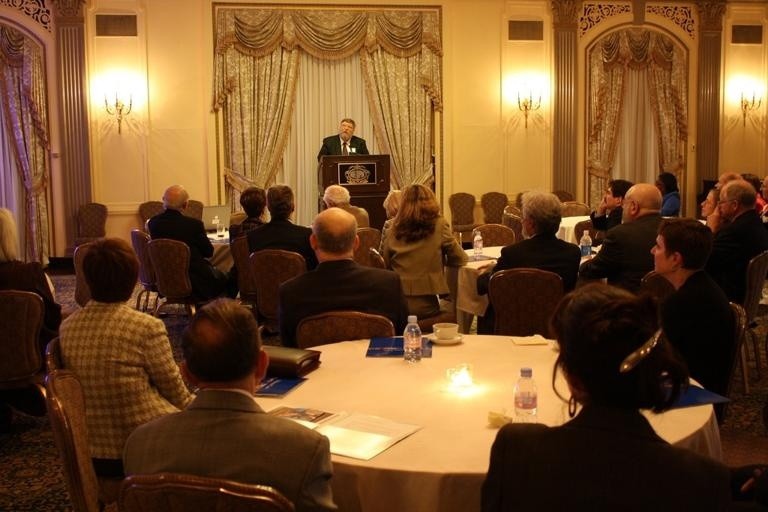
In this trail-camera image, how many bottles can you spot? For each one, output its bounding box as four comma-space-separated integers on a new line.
403, 315, 422, 365
474, 231, 484, 259
580, 231, 592, 262
514, 368, 539, 425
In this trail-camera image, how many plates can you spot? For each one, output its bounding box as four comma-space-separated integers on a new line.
427, 332, 464, 344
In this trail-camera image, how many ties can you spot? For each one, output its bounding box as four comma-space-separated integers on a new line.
342, 142, 348, 155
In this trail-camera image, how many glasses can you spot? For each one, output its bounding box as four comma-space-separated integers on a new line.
718, 199, 733, 207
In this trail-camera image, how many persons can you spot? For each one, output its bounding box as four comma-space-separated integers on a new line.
56, 170, 470, 511
0, 205, 64, 354
317, 118, 369, 162
476, 170, 766, 512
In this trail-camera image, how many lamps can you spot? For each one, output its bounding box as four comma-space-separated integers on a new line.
105, 77, 133, 133
741, 87, 762, 127
517, 77, 541, 128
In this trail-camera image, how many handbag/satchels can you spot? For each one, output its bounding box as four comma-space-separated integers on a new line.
135, 307, 192, 356
261, 346, 323, 376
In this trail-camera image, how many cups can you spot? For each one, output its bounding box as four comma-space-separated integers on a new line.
432, 323, 459, 338
216, 224, 225, 237
